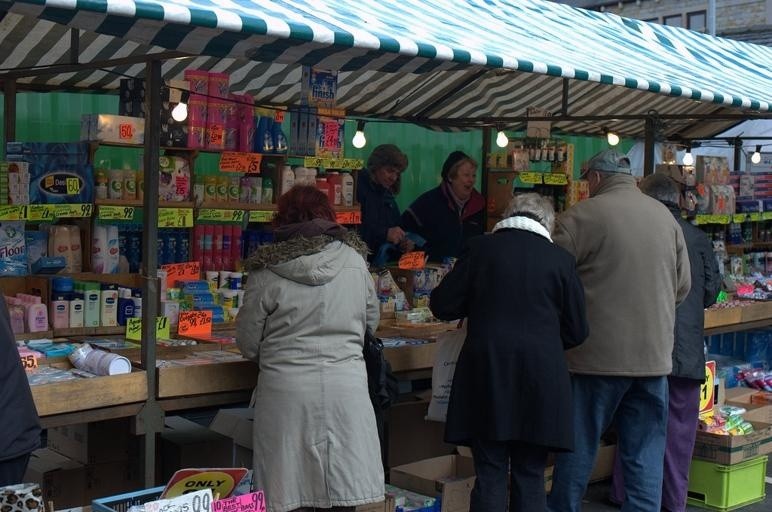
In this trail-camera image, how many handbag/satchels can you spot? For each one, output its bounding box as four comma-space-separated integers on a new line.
362, 320, 403, 416
420, 314, 480, 424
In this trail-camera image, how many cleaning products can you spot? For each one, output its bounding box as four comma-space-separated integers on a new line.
0, 220, 65, 276
159, 155, 191, 202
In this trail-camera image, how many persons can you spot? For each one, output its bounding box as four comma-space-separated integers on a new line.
428, 193, 589, 512
352, 144, 428, 271
608, 174, 722, 512
399, 151, 487, 263
551, 148, 692, 512
234, 184, 386, 512
0, 286, 45, 486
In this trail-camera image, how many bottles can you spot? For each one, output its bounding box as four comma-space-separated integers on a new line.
120, 226, 243, 272
205, 271, 246, 323
281, 163, 353, 207
97, 168, 273, 204
6, 277, 142, 335
742, 246, 772, 275
39, 224, 121, 274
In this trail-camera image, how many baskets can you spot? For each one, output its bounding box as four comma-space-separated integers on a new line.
90, 483, 167, 511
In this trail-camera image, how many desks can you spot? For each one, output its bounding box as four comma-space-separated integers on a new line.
0, 277, 770, 493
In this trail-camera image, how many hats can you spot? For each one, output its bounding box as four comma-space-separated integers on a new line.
366, 141, 410, 175
439, 149, 470, 184
576, 147, 634, 181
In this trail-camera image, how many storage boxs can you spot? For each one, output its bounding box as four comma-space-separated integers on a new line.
17, 372, 772, 512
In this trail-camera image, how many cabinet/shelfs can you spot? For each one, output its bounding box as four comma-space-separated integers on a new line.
1, 132, 372, 343
483, 136, 575, 237
629, 172, 771, 297
